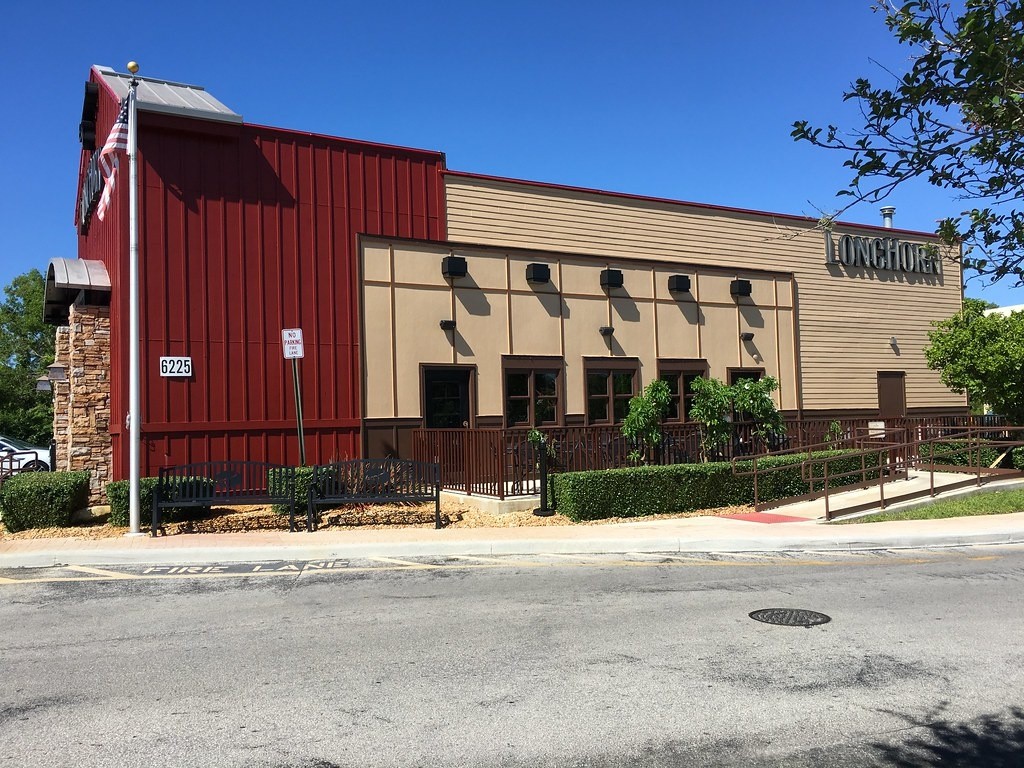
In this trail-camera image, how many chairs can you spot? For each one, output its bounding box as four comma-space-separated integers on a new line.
148, 459, 298, 534
307, 456, 442, 530
488, 432, 704, 495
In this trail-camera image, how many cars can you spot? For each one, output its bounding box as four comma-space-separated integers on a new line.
0, 437, 54, 477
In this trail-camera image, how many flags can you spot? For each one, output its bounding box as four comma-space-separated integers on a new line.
96, 92, 131, 220
